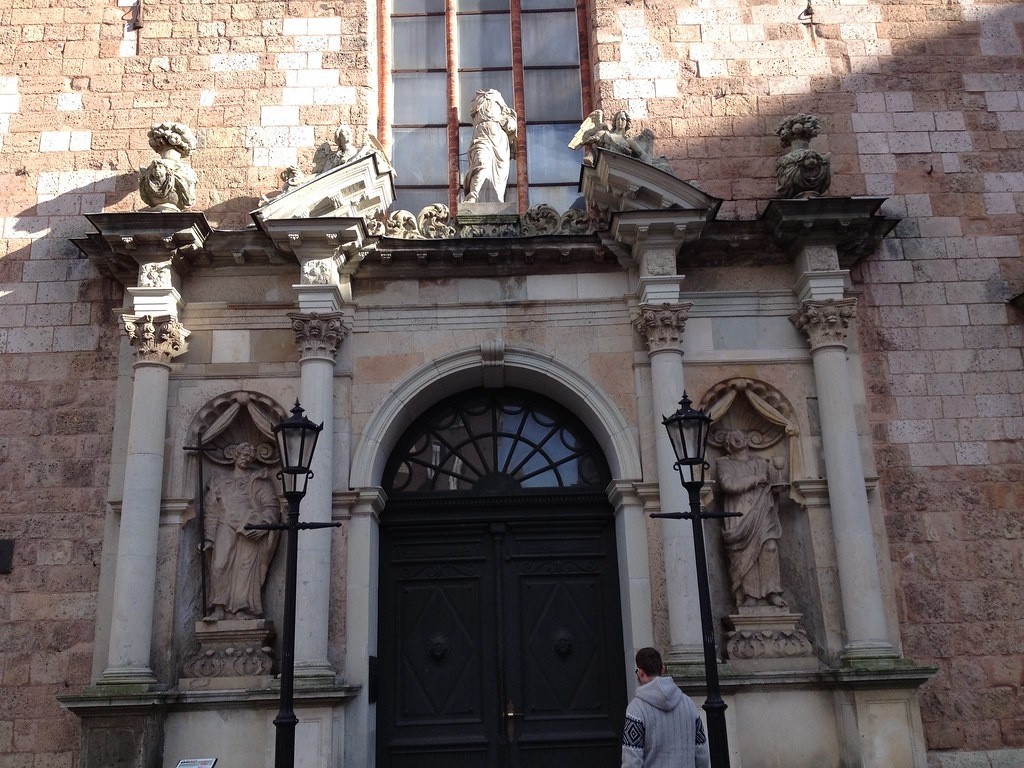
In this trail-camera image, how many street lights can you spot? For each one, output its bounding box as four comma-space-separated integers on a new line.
649, 391, 744, 767
244, 398, 342, 767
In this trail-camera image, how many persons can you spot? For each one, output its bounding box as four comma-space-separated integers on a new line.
290, 124, 396, 231
145, 160, 167, 184
622, 647, 710, 768
463, 89, 517, 203
716, 430, 790, 607
197, 442, 280, 622
584, 110, 643, 167
798, 149, 820, 177
